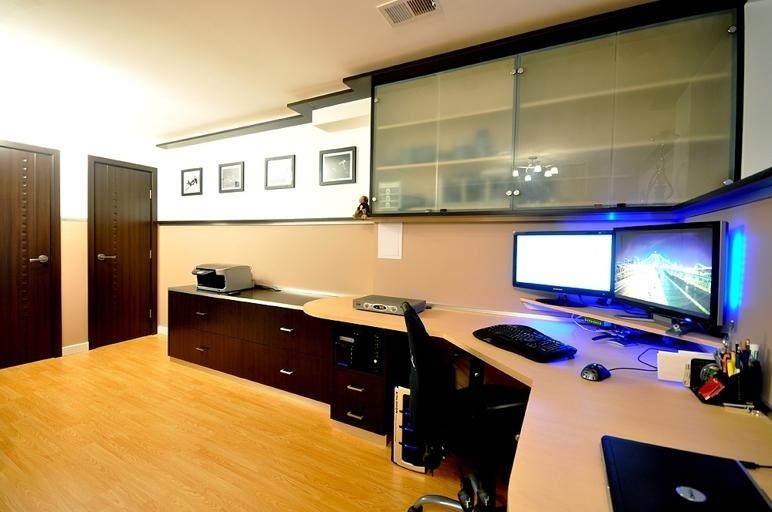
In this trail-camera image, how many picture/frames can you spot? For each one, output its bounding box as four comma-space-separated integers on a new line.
319, 145, 356, 186
180, 167, 202, 196
218, 161, 244, 193
264, 154, 295, 190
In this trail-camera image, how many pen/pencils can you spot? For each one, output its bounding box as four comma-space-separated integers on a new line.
720, 320, 759, 378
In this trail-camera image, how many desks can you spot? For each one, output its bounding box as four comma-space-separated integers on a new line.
303, 295, 771, 511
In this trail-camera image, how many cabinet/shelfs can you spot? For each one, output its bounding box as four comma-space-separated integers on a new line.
168, 282, 333, 405
369, 9, 738, 217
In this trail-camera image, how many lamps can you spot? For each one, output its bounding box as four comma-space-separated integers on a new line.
512, 155, 559, 182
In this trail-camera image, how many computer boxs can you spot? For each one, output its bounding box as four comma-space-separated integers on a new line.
393, 378, 430, 474
498, 388, 530, 483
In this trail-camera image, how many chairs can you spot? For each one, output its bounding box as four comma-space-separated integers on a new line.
400, 301, 529, 511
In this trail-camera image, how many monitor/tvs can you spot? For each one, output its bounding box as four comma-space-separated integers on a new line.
612, 221, 726, 329
513, 231, 614, 307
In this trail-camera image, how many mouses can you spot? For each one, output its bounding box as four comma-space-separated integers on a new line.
581, 363, 610, 381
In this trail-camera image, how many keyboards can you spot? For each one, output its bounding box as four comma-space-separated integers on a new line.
473, 324, 577, 362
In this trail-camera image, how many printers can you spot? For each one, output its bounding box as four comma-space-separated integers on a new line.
192, 263, 253, 293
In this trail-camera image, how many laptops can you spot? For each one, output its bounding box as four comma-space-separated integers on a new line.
601, 436, 772, 512
